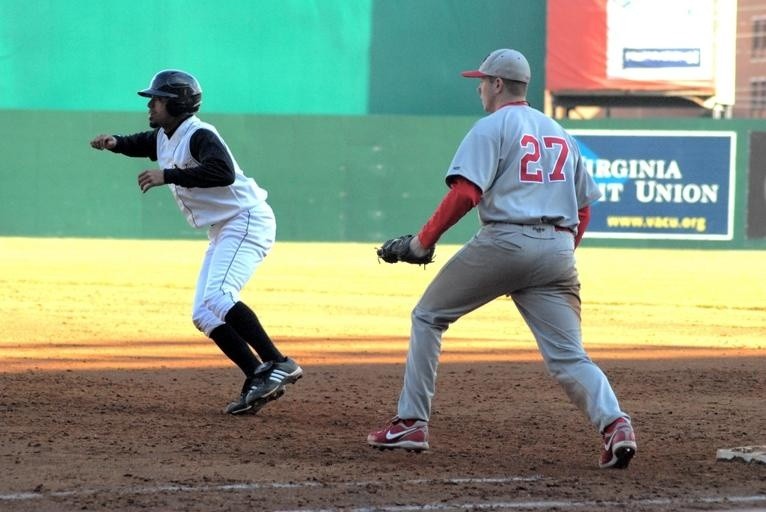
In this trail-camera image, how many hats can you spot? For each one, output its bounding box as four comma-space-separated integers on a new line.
137, 70, 201, 116
461, 49, 530, 85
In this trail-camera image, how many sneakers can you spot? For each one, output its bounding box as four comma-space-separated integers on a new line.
245, 354, 303, 405
367, 415, 430, 450
226, 370, 286, 415
598, 417, 637, 469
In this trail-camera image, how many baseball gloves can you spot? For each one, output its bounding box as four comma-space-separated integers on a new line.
377, 235, 434, 263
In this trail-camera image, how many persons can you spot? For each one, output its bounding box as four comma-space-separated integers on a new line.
366, 48, 637, 470
87, 71, 305, 416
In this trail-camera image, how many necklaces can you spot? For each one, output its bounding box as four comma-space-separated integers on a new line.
521, 99, 532, 107
